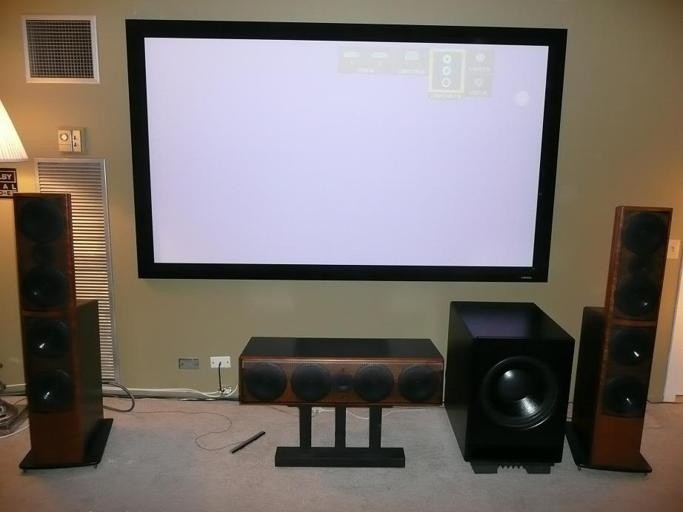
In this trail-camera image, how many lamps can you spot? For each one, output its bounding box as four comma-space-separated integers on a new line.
1, 98, 29, 162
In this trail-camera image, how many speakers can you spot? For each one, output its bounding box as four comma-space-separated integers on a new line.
571, 205, 675, 469
444, 301, 575, 476
239, 335, 443, 408
13, 191, 104, 465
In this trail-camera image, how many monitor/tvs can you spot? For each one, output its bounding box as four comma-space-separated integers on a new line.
122, 17, 569, 285
